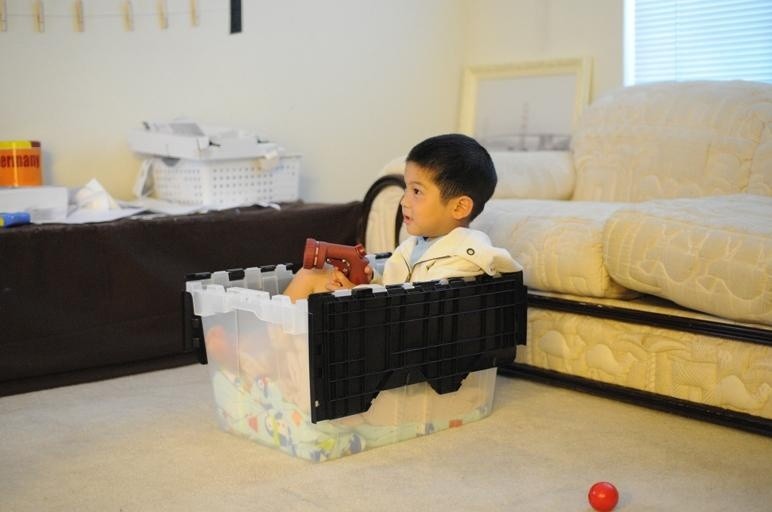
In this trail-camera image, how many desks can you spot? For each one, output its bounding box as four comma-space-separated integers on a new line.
1, 200, 366, 398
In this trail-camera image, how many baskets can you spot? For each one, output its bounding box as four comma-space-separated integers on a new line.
154, 153, 303, 206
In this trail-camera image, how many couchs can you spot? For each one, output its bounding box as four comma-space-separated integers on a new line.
362, 78, 772, 442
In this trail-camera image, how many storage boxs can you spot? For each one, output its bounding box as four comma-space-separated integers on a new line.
182, 259, 529, 464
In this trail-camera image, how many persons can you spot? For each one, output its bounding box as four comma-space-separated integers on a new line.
280, 131, 524, 306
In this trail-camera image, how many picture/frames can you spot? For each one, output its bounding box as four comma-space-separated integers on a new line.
458, 54, 594, 152
